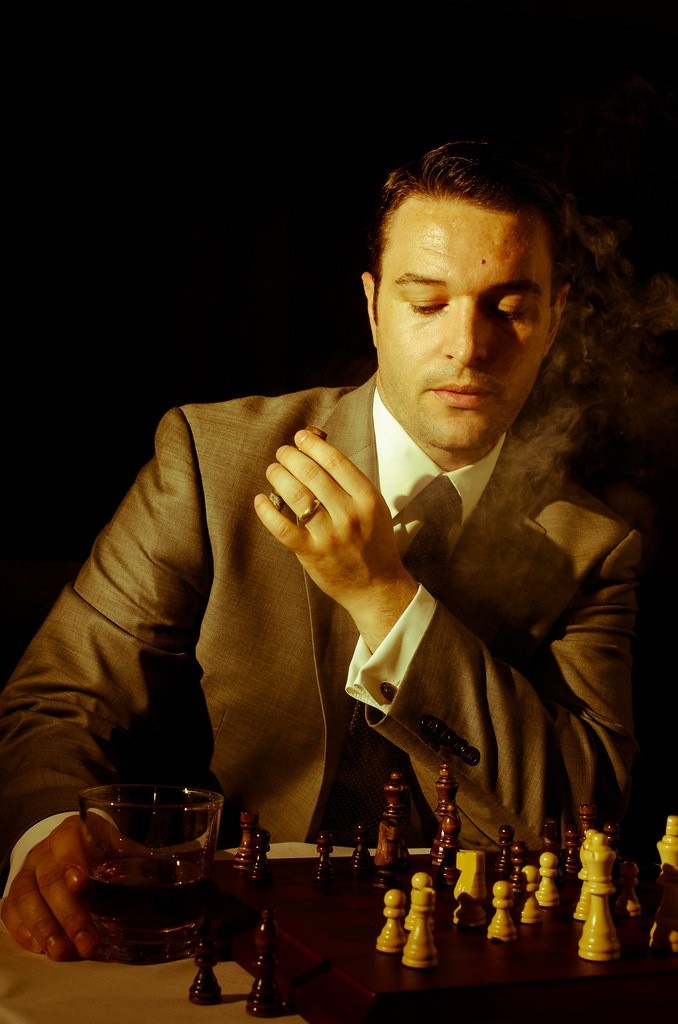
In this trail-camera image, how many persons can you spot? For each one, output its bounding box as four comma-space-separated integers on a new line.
0, 143, 643, 961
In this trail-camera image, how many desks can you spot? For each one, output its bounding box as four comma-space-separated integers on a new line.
0, 842, 432, 1024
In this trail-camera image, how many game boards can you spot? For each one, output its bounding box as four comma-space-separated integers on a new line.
188, 847, 678, 1024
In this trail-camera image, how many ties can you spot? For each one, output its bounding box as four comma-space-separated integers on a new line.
321, 476, 463, 849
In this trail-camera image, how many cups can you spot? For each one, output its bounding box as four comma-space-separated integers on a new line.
78, 783, 224, 965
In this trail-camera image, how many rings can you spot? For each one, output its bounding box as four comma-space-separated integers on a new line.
299, 499, 320, 524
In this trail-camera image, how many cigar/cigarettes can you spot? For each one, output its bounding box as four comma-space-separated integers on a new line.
269, 426, 328, 512
